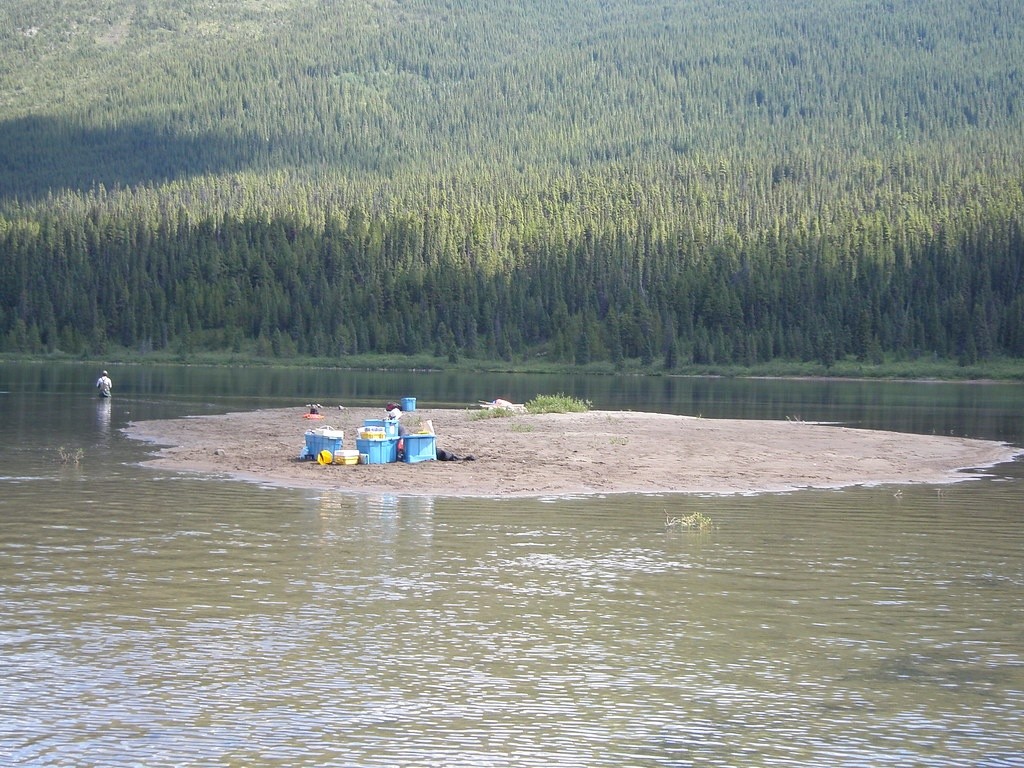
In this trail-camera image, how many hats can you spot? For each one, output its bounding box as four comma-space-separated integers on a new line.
103, 371, 107, 375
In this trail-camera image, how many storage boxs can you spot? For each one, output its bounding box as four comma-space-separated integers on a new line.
358, 454, 370, 465
365, 419, 400, 436
356, 438, 388, 466
386, 436, 401, 463
401, 397, 417, 411
316, 429, 343, 436
334, 456, 359, 466
305, 434, 344, 461
310, 409, 317, 414
334, 450, 360, 457
401, 435, 438, 464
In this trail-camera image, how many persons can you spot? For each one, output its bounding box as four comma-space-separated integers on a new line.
96, 370, 112, 397
